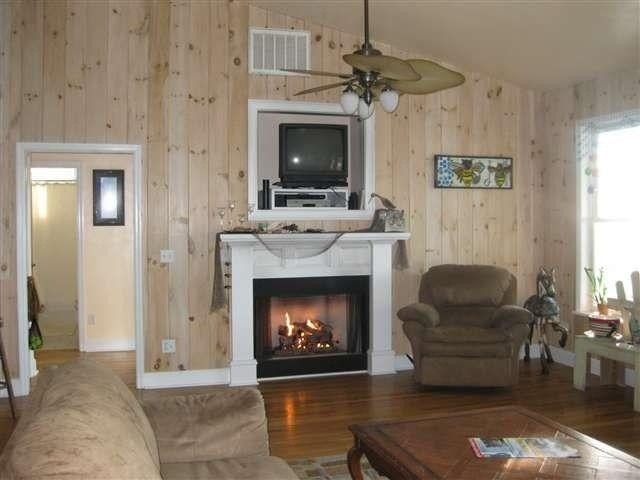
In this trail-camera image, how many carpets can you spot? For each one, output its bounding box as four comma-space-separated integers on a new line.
287, 453, 391, 480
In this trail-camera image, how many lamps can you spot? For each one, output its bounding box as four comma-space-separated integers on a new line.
340, 73, 398, 120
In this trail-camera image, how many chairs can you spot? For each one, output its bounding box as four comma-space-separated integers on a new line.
397, 272, 534, 390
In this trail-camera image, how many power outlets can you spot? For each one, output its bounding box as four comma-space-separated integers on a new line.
162, 339, 177, 354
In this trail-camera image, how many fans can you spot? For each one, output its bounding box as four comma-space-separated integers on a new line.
281, 0, 465, 96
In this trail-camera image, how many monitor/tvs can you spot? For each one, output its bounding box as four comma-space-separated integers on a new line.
279, 123, 348, 182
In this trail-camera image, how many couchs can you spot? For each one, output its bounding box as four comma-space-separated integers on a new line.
1, 363, 300, 480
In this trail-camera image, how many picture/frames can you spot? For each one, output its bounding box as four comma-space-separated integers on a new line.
92, 170, 124, 226
435, 154, 513, 189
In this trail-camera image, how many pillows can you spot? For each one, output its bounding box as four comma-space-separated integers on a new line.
428, 265, 511, 307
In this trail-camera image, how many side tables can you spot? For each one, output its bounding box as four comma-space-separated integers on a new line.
573, 335, 640, 412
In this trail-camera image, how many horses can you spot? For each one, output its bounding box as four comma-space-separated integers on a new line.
522, 264, 570, 372
